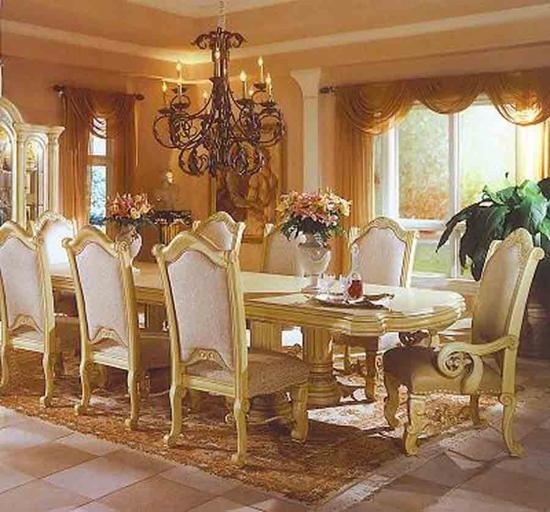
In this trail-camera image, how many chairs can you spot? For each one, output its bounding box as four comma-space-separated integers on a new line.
189, 209, 247, 257
150, 227, 313, 469
381, 225, 545, 459
26, 209, 80, 264
1, 218, 111, 408
258, 222, 332, 277
60, 224, 172, 430
330, 215, 421, 402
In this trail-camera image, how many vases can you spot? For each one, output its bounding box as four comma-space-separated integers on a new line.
292, 233, 333, 298
113, 222, 145, 275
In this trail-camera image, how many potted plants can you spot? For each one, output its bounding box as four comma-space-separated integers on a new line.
435, 171, 550, 360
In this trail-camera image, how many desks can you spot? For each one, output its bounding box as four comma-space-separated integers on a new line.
48, 257, 466, 409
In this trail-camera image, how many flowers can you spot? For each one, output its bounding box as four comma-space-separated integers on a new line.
103, 192, 156, 230
274, 187, 353, 242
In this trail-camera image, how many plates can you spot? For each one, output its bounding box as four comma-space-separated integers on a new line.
313, 291, 395, 309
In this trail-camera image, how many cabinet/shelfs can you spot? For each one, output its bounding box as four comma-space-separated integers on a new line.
0, 96, 65, 235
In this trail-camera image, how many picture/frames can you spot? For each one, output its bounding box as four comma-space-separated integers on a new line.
211, 124, 287, 243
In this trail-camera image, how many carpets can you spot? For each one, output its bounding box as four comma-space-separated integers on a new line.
0, 324, 525, 505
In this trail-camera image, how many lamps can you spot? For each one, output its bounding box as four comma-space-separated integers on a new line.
153, 0, 286, 179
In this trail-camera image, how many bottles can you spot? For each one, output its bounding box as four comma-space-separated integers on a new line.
348, 276, 363, 298
